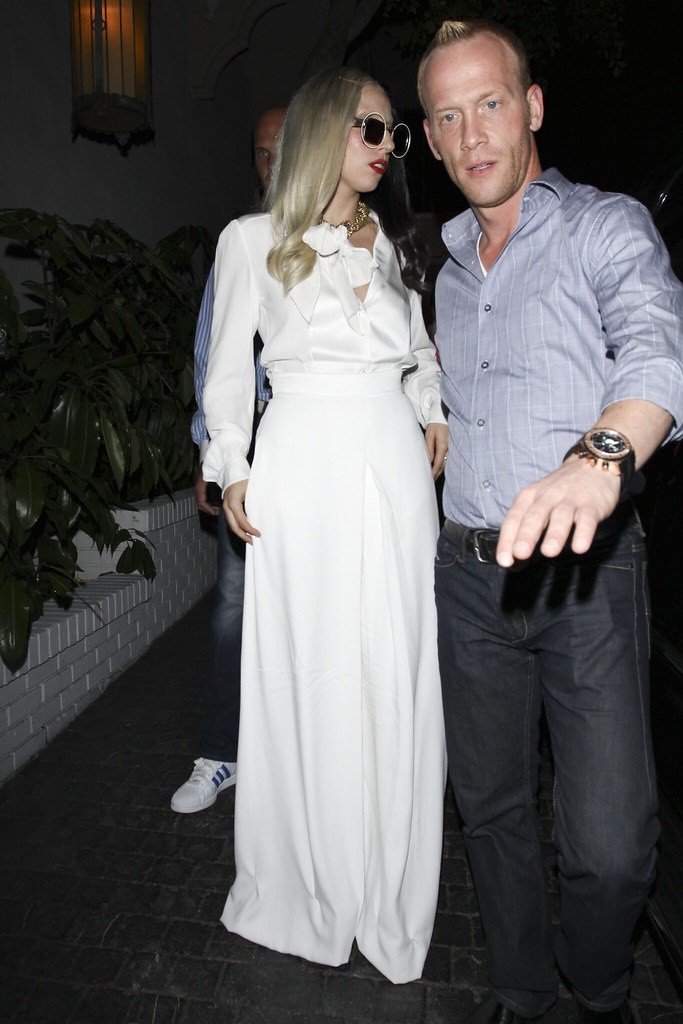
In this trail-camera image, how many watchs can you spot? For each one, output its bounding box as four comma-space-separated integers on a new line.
563, 427, 635, 485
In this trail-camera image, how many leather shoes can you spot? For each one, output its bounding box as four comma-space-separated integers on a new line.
489, 997, 561, 1024
578, 999, 635, 1024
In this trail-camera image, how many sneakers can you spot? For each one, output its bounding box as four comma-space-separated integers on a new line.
169, 757, 236, 813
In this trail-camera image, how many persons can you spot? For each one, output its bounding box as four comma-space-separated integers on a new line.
201, 61, 451, 985
416, 19, 683, 1024
170, 96, 294, 814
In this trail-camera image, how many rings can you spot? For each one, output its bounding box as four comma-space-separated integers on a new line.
444, 457, 447, 460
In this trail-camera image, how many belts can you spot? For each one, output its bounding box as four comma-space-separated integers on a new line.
440, 504, 630, 564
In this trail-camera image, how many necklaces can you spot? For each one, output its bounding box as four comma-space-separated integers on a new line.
309, 201, 369, 243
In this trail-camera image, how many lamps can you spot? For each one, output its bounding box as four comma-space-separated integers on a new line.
67, 0, 156, 150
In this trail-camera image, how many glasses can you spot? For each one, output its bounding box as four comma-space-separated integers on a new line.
351, 112, 412, 159
